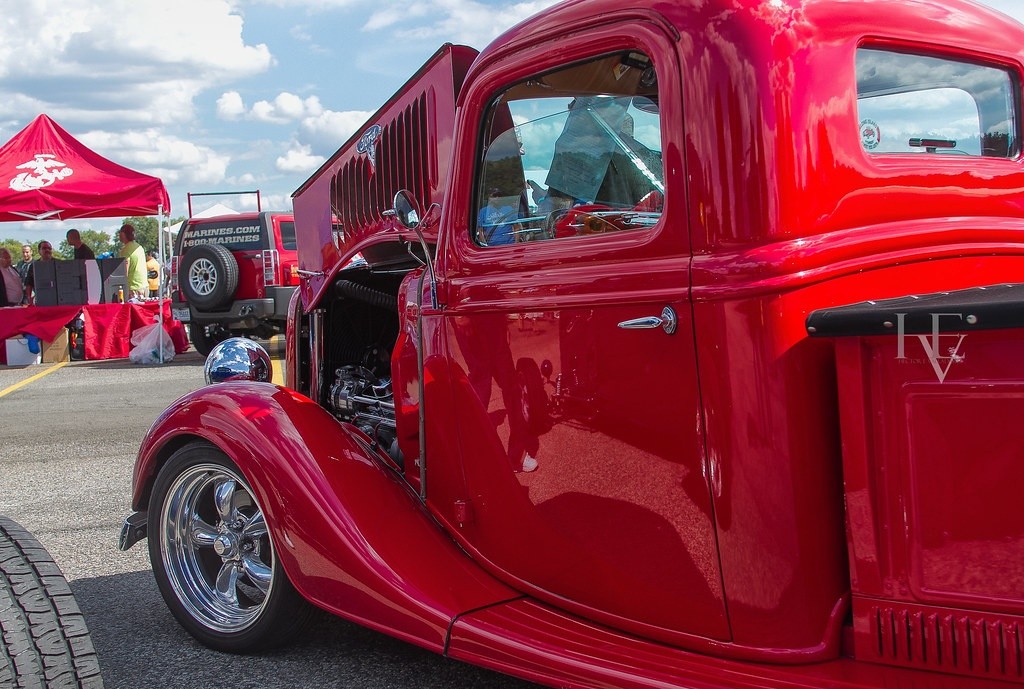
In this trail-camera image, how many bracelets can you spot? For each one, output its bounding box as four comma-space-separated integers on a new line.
535, 195, 544, 203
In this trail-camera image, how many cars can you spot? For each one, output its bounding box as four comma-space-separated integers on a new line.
118, 1, 1024, 689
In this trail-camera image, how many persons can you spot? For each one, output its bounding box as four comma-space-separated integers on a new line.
522, 101, 663, 215
476, 187, 521, 245
0, 224, 170, 308
456, 313, 538, 474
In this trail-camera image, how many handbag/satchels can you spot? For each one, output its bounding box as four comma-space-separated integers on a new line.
148, 271, 158, 279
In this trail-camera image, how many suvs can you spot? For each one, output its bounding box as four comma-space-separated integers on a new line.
172, 211, 344, 358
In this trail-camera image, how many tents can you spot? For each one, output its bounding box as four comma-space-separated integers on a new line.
164, 204, 241, 234
0, 113, 173, 364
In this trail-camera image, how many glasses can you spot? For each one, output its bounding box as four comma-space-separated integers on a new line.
41, 247, 52, 250
0, 256, 11, 261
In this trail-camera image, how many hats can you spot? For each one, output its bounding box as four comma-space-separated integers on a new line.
121, 224, 136, 240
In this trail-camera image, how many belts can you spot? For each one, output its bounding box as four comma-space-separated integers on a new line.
8, 302, 21, 306
